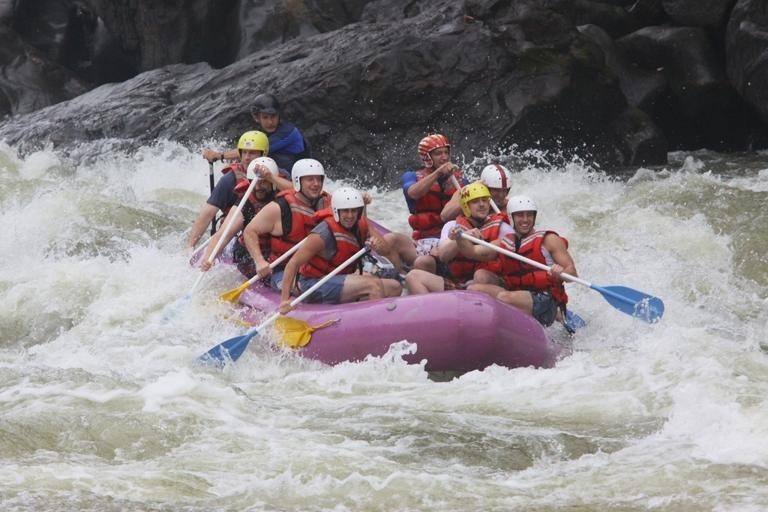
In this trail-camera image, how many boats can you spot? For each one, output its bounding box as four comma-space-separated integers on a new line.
201, 217, 565, 373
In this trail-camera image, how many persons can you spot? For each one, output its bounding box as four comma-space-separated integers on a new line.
202, 92, 307, 180
220, 151, 225, 163
240, 156, 375, 281
179, 130, 293, 253
199, 156, 290, 287
385, 130, 579, 329
278, 185, 402, 318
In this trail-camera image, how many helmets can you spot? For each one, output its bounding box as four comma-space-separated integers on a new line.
237, 130, 270, 160
246, 156, 280, 192
331, 187, 365, 223
249, 92, 281, 118
480, 163, 513, 197
458, 182, 492, 219
291, 158, 325, 197
506, 194, 538, 229
417, 134, 451, 169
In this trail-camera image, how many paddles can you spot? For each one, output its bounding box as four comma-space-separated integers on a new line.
461, 233, 664, 331
200, 238, 369, 369
